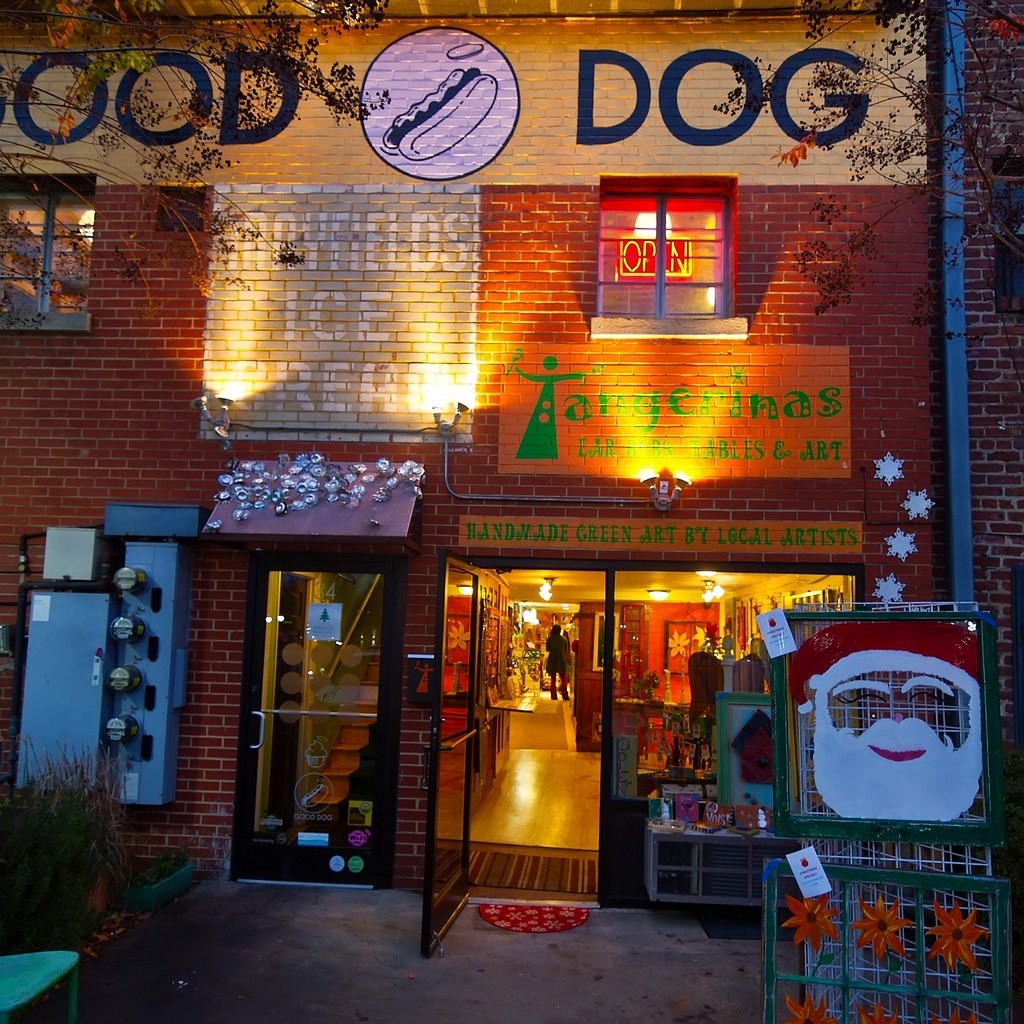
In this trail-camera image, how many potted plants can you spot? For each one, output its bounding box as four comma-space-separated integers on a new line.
127, 845, 193, 912
13, 731, 131, 914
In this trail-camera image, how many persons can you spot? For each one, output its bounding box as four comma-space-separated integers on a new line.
546, 625, 570, 701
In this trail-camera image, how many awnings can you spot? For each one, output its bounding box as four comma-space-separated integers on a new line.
201, 457, 424, 550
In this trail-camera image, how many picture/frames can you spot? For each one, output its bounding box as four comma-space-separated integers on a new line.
663, 620, 713, 675
594, 611, 619, 672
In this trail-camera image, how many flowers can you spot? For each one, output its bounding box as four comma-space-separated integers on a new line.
855, 1000, 899, 1024
850, 893, 912, 963
931, 1006, 977, 1024
781, 991, 839, 1024
924, 898, 990, 973
779, 893, 838, 954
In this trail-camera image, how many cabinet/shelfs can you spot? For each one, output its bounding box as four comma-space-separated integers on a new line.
644, 819, 800, 908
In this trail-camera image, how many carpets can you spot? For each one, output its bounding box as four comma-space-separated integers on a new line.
469, 850, 598, 894
478, 903, 591, 933
699, 912, 795, 941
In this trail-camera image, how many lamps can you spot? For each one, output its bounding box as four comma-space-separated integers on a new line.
701, 580, 724, 603
538, 578, 555, 602
647, 589, 671, 601
637, 468, 691, 511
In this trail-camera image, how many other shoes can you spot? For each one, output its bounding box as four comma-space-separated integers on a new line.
558, 687, 570, 700
552, 692, 557, 699
